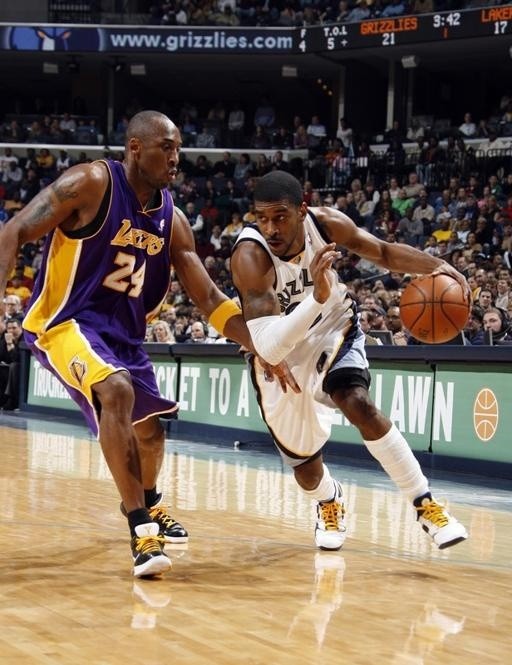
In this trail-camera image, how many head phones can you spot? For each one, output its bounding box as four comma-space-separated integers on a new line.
198, 320, 209, 336
481, 309, 512, 334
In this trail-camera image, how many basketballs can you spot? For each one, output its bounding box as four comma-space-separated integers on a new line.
399, 274, 469, 344
474, 389, 499, 441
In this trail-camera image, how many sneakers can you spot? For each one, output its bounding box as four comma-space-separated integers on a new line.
314, 479, 347, 550
130, 521, 172, 578
414, 492, 467, 550
121, 491, 190, 544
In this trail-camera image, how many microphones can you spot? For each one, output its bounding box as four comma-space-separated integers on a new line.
191, 335, 206, 340
493, 326, 511, 340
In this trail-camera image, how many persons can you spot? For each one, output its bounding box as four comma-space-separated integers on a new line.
1, 108, 136, 347
228, 169, 478, 556
132, 2, 435, 28
144, 97, 512, 346
0, 109, 303, 581
